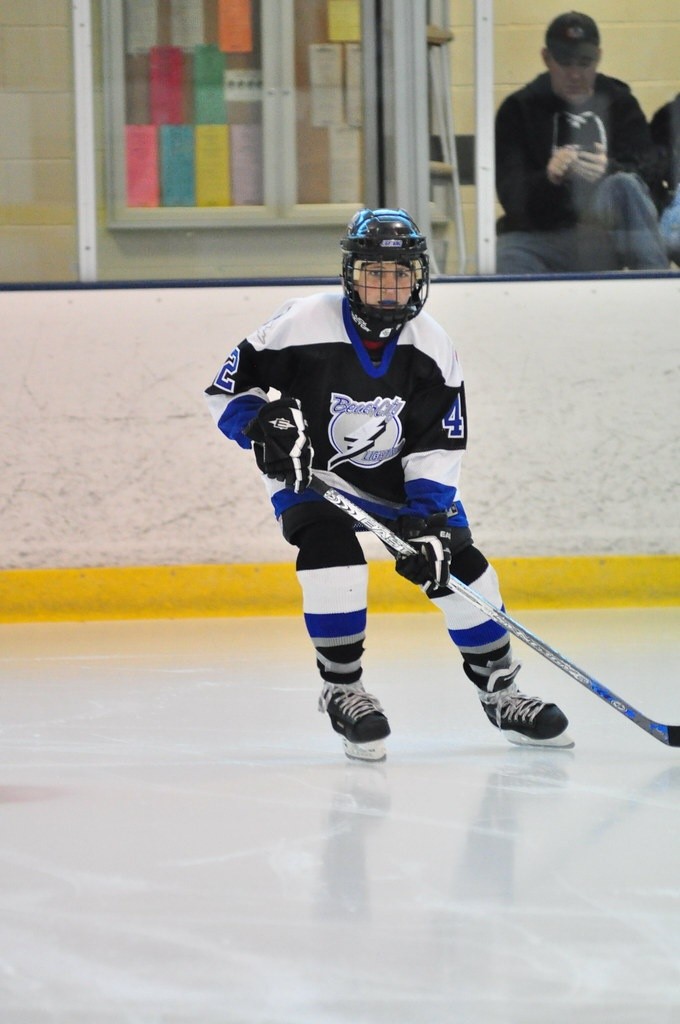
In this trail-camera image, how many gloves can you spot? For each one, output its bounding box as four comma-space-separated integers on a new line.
393, 514, 456, 597
240, 399, 314, 497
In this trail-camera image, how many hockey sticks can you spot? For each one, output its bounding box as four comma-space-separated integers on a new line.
305, 474, 678, 750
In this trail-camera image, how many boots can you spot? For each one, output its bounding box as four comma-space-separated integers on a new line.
463, 658, 577, 749
316, 678, 391, 763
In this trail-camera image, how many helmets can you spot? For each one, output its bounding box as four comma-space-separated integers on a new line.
338, 208, 431, 340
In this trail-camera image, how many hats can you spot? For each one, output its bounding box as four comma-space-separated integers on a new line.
545, 12, 599, 62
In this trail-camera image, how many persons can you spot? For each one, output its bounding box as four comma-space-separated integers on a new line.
494, 12, 680, 276
205, 210, 576, 761
